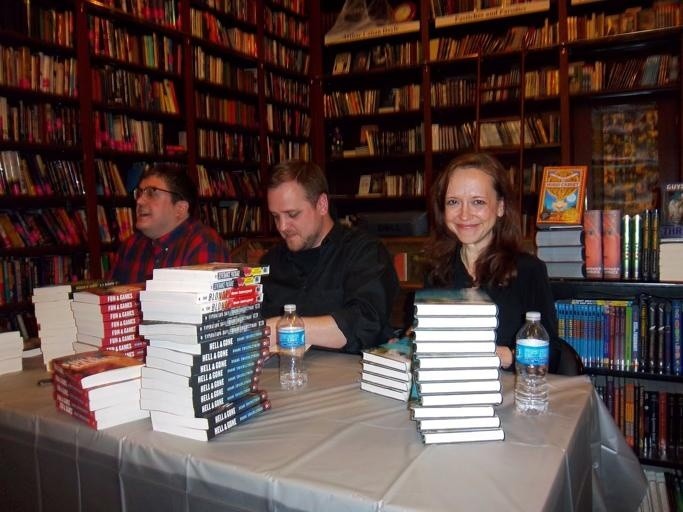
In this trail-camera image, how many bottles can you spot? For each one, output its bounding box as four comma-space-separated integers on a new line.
515, 312, 549, 416
276, 304, 306, 390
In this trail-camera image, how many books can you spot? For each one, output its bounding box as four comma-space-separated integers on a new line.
567, 0, 683, 93
587, 374, 683, 512
658, 182, 683, 282
584, 209, 659, 280
412, 289, 504, 444
1, 262, 272, 441
2, 0, 313, 111
428, 0, 561, 150
2, 202, 137, 343
554, 293, 683, 376
359, 337, 413, 400
324, 0, 424, 198
1, 98, 312, 200
534, 164, 587, 278
202, 202, 285, 266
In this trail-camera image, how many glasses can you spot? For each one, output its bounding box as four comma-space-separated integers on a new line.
133, 186, 184, 201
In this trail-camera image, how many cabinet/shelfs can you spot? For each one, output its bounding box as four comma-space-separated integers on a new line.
264, 0, 322, 168
190, 1, 270, 257
561, 0, 683, 210
546, 274, 683, 512
422, 0, 565, 237
319, 0, 425, 230
0, 0, 100, 340
85, 1, 202, 280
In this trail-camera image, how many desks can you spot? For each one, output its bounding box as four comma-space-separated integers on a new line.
1, 342, 648, 512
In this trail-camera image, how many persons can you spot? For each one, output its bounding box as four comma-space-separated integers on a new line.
103, 163, 229, 286
255, 159, 401, 361
389, 151, 558, 376
